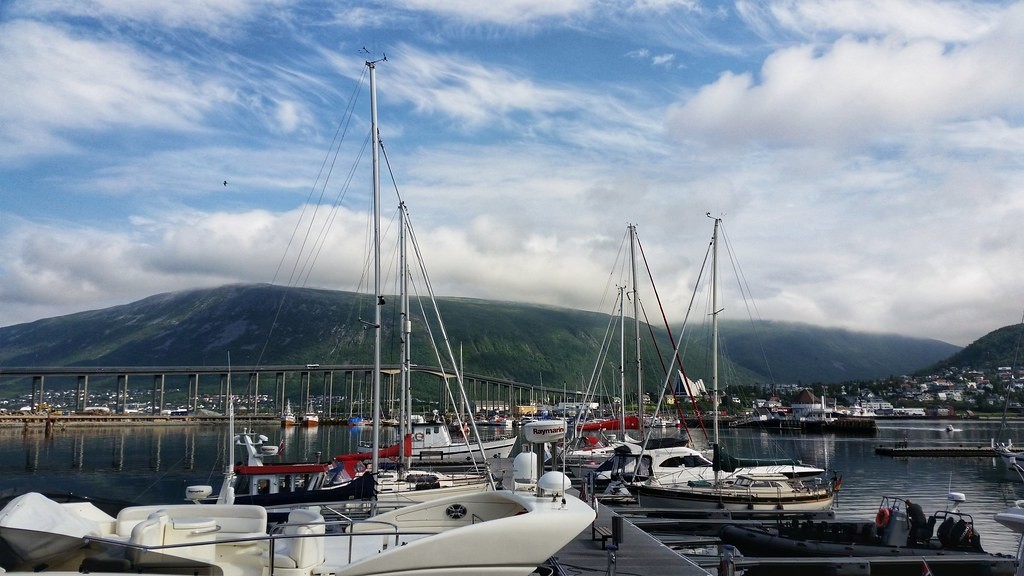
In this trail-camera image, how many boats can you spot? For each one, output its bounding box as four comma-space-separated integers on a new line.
719, 471, 986, 555
946, 424, 952, 432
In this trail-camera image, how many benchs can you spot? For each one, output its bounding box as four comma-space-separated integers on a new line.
115, 504, 267, 546
592, 526, 614, 550
258, 509, 326, 576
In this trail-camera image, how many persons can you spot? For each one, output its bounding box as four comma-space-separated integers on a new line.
906, 500, 926, 536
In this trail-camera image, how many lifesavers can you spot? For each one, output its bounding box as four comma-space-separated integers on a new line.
414, 432, 425, 441
875, 507, 889, 528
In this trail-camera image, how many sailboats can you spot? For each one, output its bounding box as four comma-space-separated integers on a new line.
0, 54, 595, 575
626, 211, 837, 520
561, 219, 826, 478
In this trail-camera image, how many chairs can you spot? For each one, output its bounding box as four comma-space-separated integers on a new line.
920, 516, 966, 547
787, 518, 878, 544
90, 509, 169, 553
